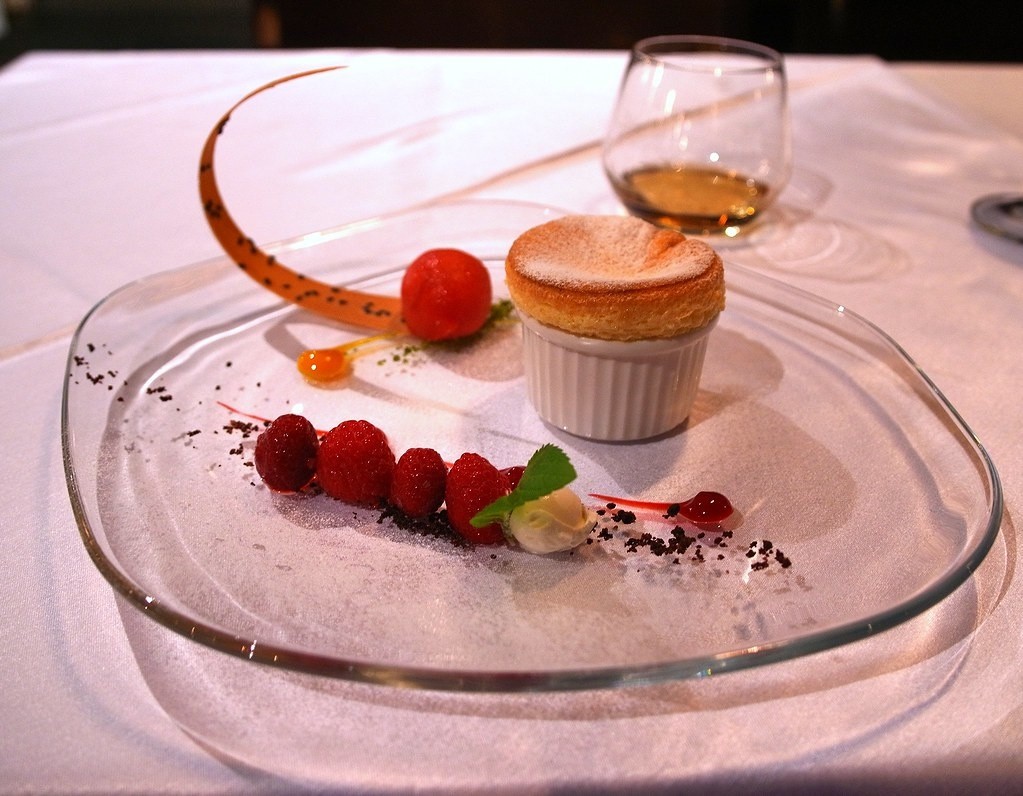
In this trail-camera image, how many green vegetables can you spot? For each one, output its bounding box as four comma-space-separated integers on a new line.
469, 444, 578, 541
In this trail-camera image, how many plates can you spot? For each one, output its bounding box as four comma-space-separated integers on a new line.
63, 198, 1004, 693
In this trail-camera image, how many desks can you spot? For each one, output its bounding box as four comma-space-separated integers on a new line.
2, 49, 1022, 796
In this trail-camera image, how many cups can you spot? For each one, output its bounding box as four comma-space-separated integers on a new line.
603, 36, 794, 236
509, 295, 721, 441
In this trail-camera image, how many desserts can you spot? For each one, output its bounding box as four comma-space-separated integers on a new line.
197, 66, 510, 343
506, 214, 725, 443
